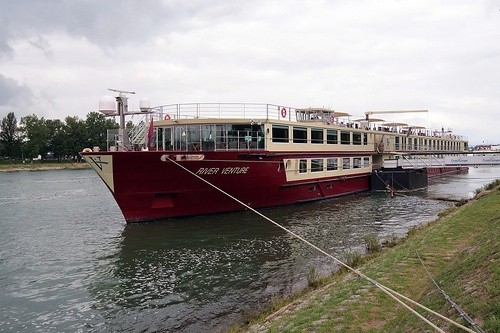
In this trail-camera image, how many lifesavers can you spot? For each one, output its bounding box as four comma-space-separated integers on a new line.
281, 108, 286, 117
164, 115, 170, 120
330, 116, 335, 122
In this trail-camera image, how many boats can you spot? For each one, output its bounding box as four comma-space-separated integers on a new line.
472, 144, 500, 151
81, 86, 470, 222
